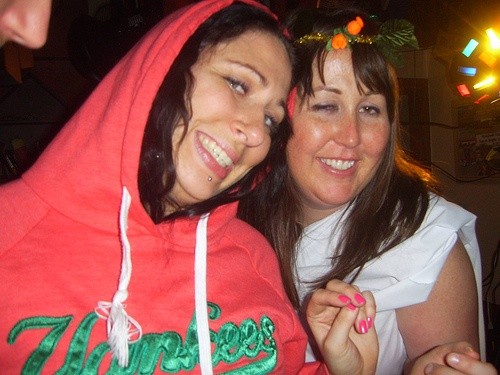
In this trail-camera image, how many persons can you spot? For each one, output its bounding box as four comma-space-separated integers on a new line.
237, 6, 500, 375
0, 0, 379, 375
0, 0, 51, 49
